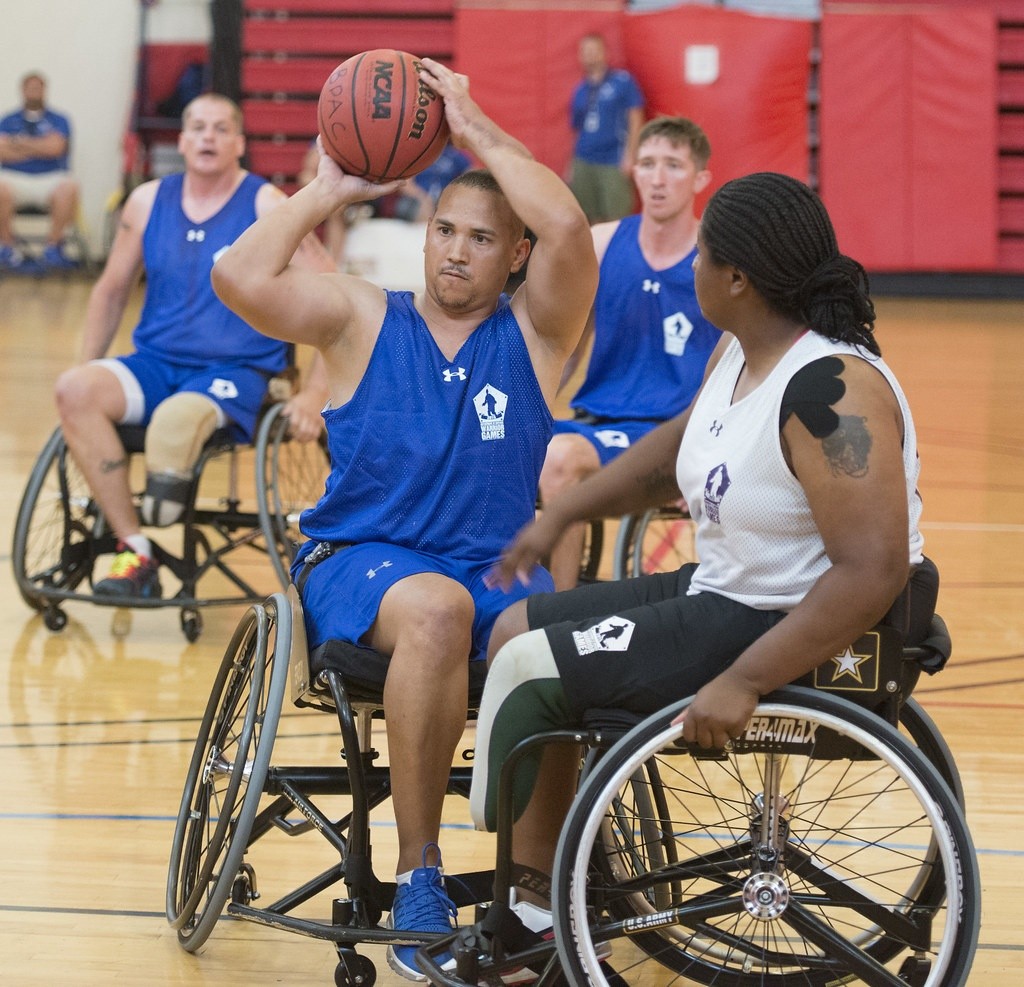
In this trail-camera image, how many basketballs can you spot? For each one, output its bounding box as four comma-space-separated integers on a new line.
314, 45, 454, 185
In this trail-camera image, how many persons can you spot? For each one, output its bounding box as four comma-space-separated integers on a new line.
211, 57, 601, 982
295, 133, 473, 262
450, 172, 922, 967
0, 75, 82, 267
53, 91, 337, 598
537, 115, 713, 593
564, 31, 646, 228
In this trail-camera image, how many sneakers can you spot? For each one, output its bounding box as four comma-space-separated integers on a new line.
95, 540, 161, 600
386, 843, 460, 982
427, 887, 612, 987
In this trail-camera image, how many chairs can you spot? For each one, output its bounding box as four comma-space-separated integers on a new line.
9, 204, 96, 277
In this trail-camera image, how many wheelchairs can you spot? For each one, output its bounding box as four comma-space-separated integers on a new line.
581, 505, 694, 585
12, 401, 334, 644
165, 593, 986, 987
